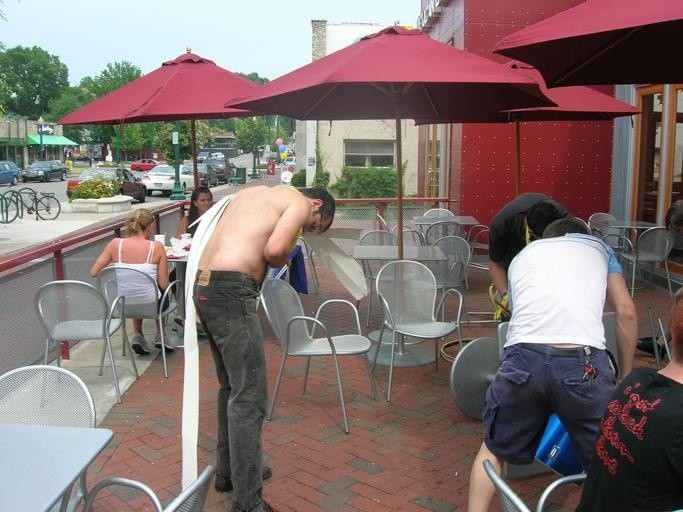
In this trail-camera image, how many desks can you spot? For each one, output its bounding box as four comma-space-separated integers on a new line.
411, 216, 480, 286
1, 423, 114, 512
353, 244, 449, 345
588, 219, 667, 283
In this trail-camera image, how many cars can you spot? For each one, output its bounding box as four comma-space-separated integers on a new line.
66, 167, 146, 204
21, 160, 67, 183
0, 161, 21, 186
131, 159, 161, 171
189, 164, 219, 187
142, 163, 201, 196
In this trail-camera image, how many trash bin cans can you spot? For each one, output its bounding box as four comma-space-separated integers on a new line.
66, 160, 73, 169
236, 168, 246, 184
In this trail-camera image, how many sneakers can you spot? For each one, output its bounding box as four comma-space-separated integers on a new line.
215, 463, 271, 492
637, 335, 667, 357
154, 332, 174, 352
132, 332, 150, 355
233, 501, 277, 512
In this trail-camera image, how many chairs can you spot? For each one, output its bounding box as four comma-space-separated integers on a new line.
83, 465, 218, 512
97, 266, 184, 378
350, 208, 674, 300
34, 279, 140, 408
258, 277, 380, 433
480, 457, 589, 512
372, 258, 464, 403
0, 364, 97, 512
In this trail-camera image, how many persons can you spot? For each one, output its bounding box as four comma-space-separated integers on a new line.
91, 208, 174, 354
573, 287, 683, 512
192, 185, 335, 512
489, 193, 572, 323
468, 220, 638, 512
168, 187, 213, 304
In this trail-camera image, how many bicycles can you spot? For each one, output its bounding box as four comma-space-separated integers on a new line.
0, 190, 61, 223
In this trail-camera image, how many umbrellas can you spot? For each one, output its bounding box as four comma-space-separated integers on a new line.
224, 15, 559, 313
492, 0, 683, 90
414, 60, 641, 198
56, 42, 265, 188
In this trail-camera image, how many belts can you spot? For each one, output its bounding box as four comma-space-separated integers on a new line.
522, 342, 596, 357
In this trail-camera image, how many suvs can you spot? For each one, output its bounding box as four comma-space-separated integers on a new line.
201, 158, 234, 183
197, 151, 225, 163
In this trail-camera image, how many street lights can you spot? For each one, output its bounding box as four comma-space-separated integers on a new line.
38, 116, 44, 159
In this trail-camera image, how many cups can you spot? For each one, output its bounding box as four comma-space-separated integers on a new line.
173, 248, 185, 254
153, 235, 165, 248
180, 234, 191, 240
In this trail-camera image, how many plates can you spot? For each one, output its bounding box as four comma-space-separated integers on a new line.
173, 254, 184, 257
